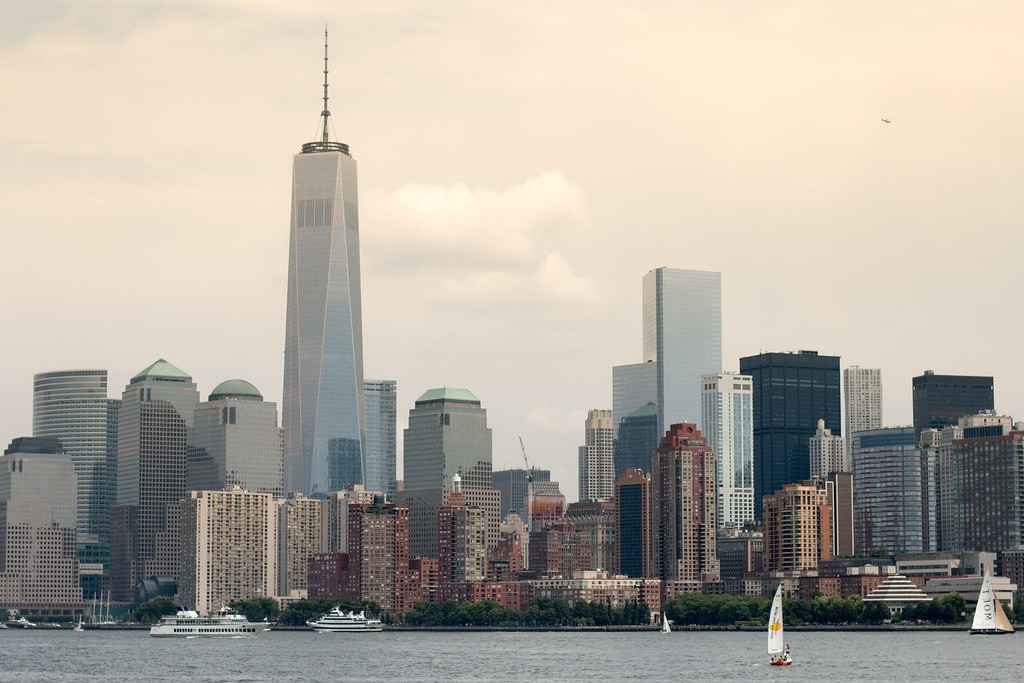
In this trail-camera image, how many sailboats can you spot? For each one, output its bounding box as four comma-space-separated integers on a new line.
661, 612, 672, 634
767, 584, 793, 666
74, 614, 85, 633
964, 568, 1017, 635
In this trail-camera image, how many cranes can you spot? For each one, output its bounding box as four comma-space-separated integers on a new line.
518, 435, 535, 530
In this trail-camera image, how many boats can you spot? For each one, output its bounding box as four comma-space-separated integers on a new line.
149, 608, 271, 638
307, 606, 385, 632
